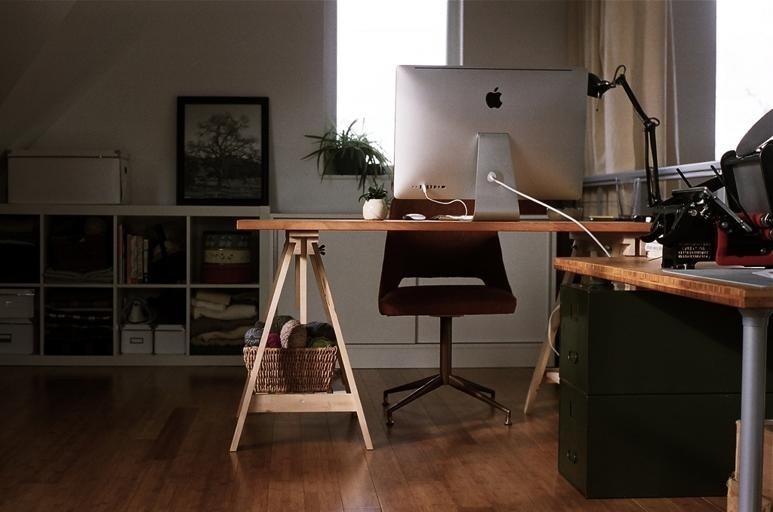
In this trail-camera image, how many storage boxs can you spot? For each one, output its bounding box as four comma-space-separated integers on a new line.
735, 418, 773, 500
0, 323, 34, 355
120, 324, 153, 354
154, 324, 186, 355
205, 248, 252, 284
0, 288, 35, 318
7, 146, 132, 204
726, 474, 773, 511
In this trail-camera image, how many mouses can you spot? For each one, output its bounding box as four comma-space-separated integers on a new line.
402, 213, 426, 221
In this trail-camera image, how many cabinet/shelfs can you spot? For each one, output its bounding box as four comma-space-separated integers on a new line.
0, 203, 273, 367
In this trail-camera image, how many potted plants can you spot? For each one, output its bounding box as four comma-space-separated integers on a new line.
299, 118, 392, 194
357, 183, 389, 220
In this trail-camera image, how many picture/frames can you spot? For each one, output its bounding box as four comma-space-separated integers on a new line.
175, 94, 270, 206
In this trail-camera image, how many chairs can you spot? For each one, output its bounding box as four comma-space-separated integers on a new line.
378, 198, 517, 425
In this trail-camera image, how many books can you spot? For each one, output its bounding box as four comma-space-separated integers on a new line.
116, 222, 153, 285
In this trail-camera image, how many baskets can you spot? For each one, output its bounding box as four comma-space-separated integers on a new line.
242, 342, 339, 394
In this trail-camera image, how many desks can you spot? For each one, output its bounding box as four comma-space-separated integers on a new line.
553, 256, 773, 512
227, 219, 654, 452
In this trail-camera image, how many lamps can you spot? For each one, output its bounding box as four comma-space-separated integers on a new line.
588, 64, 664, 208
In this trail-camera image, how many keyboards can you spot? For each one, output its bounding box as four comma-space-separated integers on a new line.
430, 213, 550, 220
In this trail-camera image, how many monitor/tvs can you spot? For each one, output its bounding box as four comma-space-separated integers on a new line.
391, 63, 590, 222
